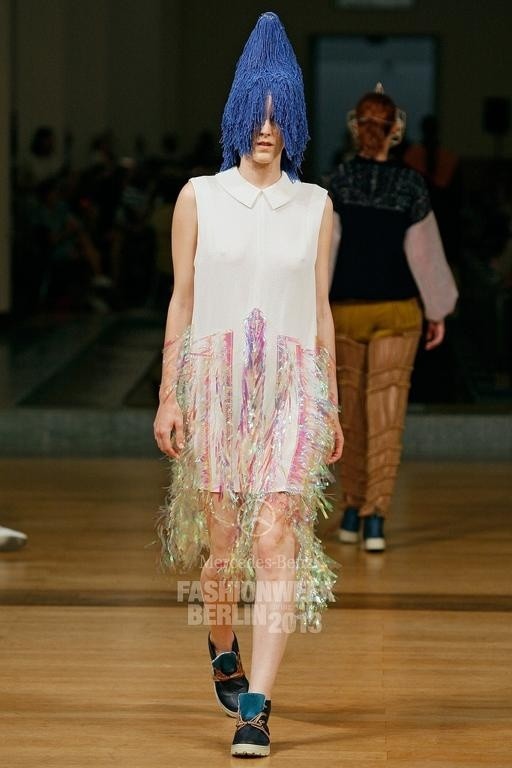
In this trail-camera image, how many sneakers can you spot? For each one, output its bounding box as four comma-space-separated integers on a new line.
207, 628, 249, 718
230, 692, 271, 758
337, 506, 360, 543
362, 515, 385, 550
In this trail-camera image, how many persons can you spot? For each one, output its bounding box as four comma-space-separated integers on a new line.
7, 110, 512, 416
320, 92, 459, 551
151, 77, 351, 757
0, 525, 29, 556
